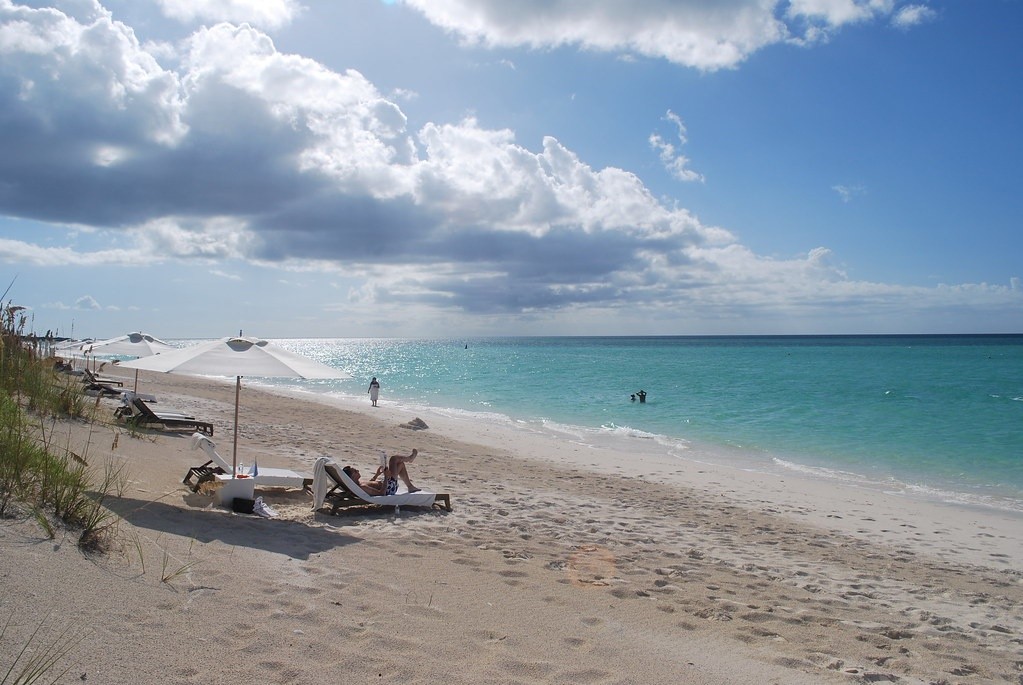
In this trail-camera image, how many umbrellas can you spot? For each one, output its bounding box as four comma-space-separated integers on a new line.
113, 328, 353, 479
48, 332, 176, 396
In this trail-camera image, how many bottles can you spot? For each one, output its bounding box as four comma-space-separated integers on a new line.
395, 505, 399, 516
238, 463, 243, 475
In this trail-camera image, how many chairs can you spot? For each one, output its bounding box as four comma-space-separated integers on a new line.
84, 368, 214, 436
182, 432, 314, 495
323, 460, 450, 515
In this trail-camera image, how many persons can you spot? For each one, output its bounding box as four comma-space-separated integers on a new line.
636, 390, 647, 402
630, 394, 636, 400
368, 376, 380, 406
343, 448, 422, 496
